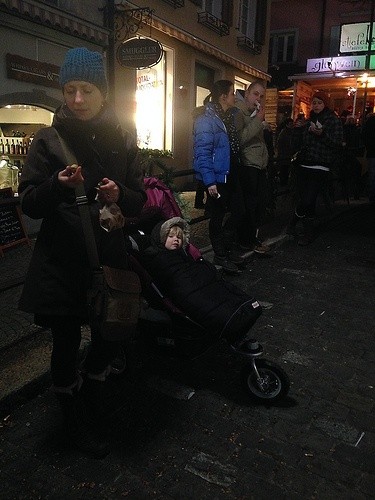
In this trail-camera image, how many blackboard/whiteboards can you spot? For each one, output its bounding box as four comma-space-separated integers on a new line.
0, 188, 32, 257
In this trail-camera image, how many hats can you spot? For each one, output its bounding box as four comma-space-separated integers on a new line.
59, 47, 107, 99
312, 91, 329, 110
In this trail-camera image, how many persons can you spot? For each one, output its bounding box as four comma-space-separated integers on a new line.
17, 46, 149, 460
143, 217, 261, 333
193, 80, 375, 271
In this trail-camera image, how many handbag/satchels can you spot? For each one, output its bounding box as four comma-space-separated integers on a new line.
86, 265, 142, 339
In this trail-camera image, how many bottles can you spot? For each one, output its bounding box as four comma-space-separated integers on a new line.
0, 138, 33, 155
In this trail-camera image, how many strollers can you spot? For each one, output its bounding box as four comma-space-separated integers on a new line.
95, 159, 289, 405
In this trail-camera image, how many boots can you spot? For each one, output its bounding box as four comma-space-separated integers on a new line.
51, 358, 129, 457
282, 207, 313, 244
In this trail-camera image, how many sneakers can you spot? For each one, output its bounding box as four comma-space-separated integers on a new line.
240, 238, 273, 254
211, 252, 244, 274
